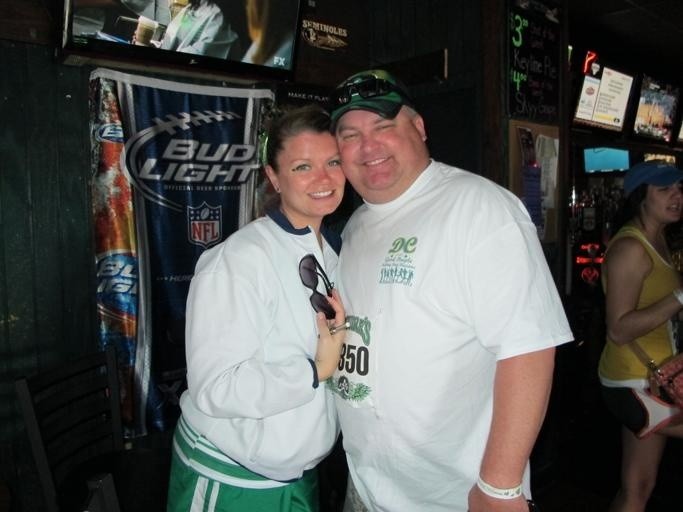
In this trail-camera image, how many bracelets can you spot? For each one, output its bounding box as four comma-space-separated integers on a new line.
477, 475, 522, 499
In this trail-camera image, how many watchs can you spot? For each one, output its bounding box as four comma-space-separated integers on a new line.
673, 289, 683, 305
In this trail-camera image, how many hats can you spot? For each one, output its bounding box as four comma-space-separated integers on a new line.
623, 158, 682, 199
328, 67, 407, 120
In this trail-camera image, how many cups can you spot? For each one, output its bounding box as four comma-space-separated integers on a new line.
133, 16, 157, 48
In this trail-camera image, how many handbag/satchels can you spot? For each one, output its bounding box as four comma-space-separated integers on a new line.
647, 351, 683, 408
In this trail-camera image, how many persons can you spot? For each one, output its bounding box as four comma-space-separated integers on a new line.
166, 108, 346, 512
332, 69, 575, 512
598, 160, 683, 512
133, 0, 293, 66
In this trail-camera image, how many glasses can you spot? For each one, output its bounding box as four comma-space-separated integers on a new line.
328, 78, 408, 105
298, 253, 337, 322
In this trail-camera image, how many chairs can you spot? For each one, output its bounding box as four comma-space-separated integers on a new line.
14, 345, 171, 511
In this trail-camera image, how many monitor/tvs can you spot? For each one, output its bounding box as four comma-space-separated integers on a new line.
566, 47, 682, 172
53, 1, 306, 87
